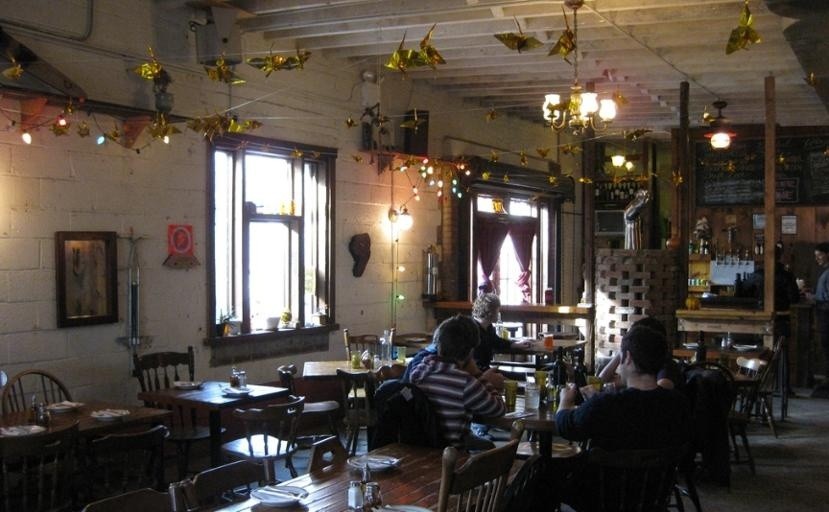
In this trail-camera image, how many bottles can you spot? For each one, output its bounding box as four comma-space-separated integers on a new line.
347, 482, 363, 509
388, 328, 398, 359
720, 337, 728, 367
364, 483, 382, 510
496, 312, 503, 338
701, 279, 705, 285
545, 373, 554, 401
38, 403, 51, 427
691, 278, 696, 286
373, 354, 379, 369
743, 272, 746, 281
688, 240, 693, 254
725, 331, 732, 349
696, 331, 706, 367
554, 346, 565, 389
705, 280, 710, 286
239, 371, 246, 388
29, 396, 38, 425
695, 278, 700, 286
699, 239, 704, 254
704, 241, 709, 255
231, 366, 239, 386
381, 331, 390, 364
574, 351, 587, 404
361, 464, 371, 494
735, 273, 741, 297
687, 279, 691, 285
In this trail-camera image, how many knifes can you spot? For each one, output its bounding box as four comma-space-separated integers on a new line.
254, 487, 310, 502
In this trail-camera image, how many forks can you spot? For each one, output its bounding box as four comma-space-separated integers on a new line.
263, 483, 307, 496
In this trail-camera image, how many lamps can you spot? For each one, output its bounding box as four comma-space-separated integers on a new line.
704, 98, 736, 149
390, 204, 413, 231
542, 0, 616, 132
611, 155, 636, 172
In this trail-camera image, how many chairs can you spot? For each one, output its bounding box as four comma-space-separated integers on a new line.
762, 335, 784, 437
736, 357, 768, 376
134, 345, 226, 473
385, 330, 433, 347
438, 418, 526, 512
1, 420, 80, 512
91, 425, 169, 499
0, 368, 75, 415
666, 462, 703, 511
337, 368, 378, 452
279, 364, 339, 439
308, 435, 350, 474
218, 394, 306, 484
81, 485, 186, 512
181, 455, 276, 512
682, 362, 754, 472
345, 329, 396, 424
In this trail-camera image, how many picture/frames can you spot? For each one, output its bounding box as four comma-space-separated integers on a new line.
53, 232, 118, 325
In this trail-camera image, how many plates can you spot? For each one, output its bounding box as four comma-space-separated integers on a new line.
734, 344, 757, 353
684, 343, 702, 351
362, 504, 437, 512
221, 387, 255, 396
346, 454, 401, 471
90, 408, 132, 421
44, 401, 84, 412
0, 423, 49, 437
248, 485, 311, 507
174, 380, 203, 389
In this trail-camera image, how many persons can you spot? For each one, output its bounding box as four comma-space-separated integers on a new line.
403, 328, 504, 392
736, 246, 799, 330
409, 318, 505, 459
500, 329, 684, 510
798, 243, 828, 397
596, 318, 684, 392
467, 293, 534, 378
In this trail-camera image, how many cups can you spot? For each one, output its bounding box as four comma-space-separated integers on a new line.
501, 329, 509, 340
525, 382, 541, 411
503, 380, 517, 410
544, 333, 554, 350
586, 376, 602, 396
396, 346, 407, 362
350, 350, 362, 369
534, 372, 548, 400
